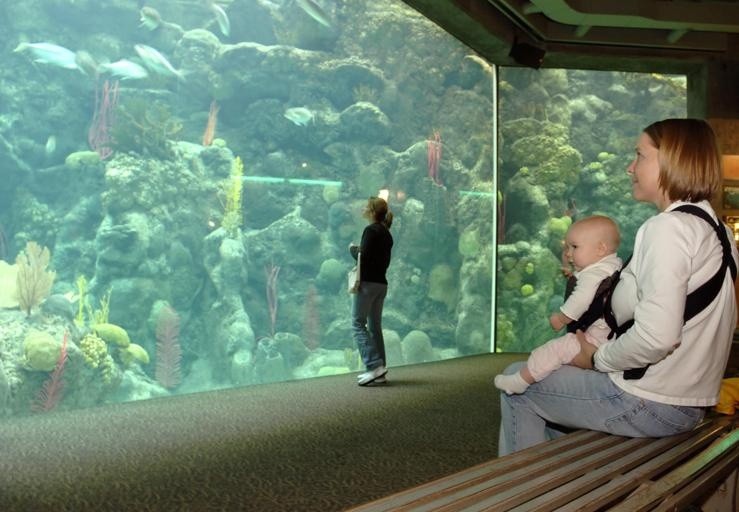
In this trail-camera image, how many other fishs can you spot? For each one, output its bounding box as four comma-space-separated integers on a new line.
284, 105, 317, 128
138, 6, 159, 31
45, 135, 58, 152
297, 1, 332, 29
13, 32, 187, 83
209, 4, 231, 36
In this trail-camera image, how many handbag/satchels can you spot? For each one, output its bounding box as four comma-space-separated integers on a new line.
347, 266, 361, 294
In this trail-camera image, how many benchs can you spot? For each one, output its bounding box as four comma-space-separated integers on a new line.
343, 409, 737, 512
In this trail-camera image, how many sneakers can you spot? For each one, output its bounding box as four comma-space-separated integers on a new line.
357, 365, 388, 386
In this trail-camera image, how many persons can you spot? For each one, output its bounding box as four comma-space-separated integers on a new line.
349, 195, 394, 387
499, 117, 738, 459
493, 214, 625, 397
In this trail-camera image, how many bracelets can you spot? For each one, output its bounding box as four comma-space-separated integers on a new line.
355, 280, 361, 283
